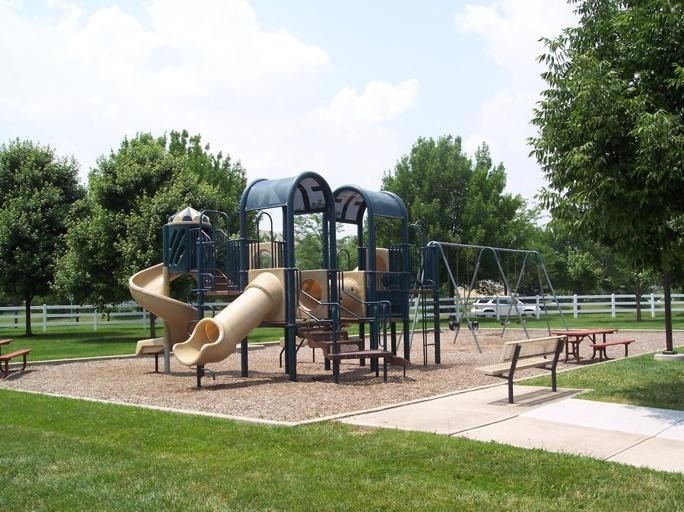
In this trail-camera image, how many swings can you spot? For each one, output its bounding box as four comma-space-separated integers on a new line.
445, 244, 460, 331
496, 249, 510, 326
465, 246, 480, 331
514, 252, 527, 325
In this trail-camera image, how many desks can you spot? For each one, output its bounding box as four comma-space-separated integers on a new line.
551, 330, 613, 363
0, 338, 14, 372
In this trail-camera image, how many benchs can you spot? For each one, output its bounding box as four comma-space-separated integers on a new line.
476, 335, 567, 403
0, 348, 32, 379
591, 339, 635, 360
568, 340, 577, 359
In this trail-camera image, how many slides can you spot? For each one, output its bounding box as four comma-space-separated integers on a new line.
172, 273, 281, 366
128, 262, 199, 358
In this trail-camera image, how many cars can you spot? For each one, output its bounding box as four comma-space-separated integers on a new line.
470, 295, 541, 316
